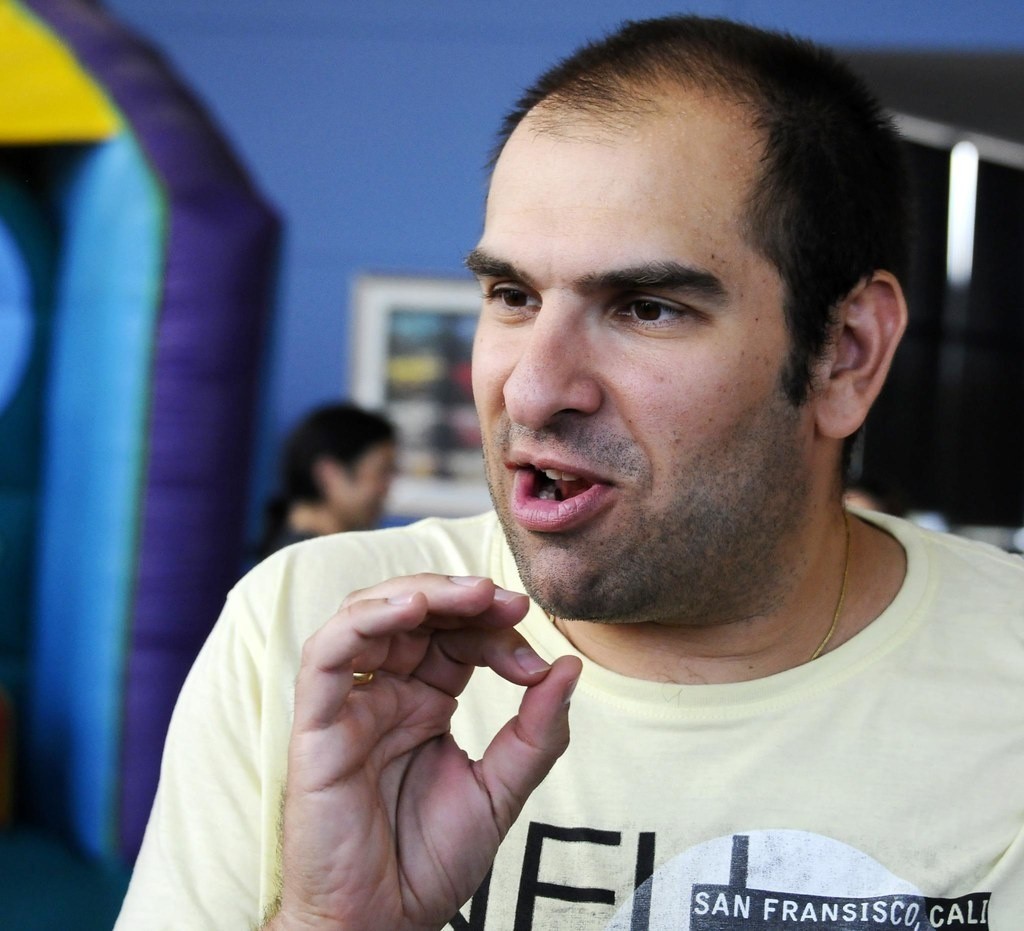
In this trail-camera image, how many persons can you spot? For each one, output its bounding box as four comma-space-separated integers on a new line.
255, 404, 395, 569
108, 13, 1023, 931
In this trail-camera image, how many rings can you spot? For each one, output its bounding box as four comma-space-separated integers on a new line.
350, 672, 375, 685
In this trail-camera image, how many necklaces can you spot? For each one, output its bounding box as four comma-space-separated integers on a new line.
544, 505, 855, 661
